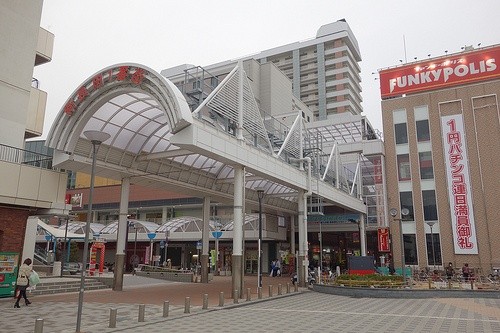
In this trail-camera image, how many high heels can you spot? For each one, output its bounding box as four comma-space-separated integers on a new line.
14, 302, 20, 308
25, 299, 33, 306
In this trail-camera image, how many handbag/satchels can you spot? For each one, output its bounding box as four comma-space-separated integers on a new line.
30, 270, 40, 285
277, 269, 281, 275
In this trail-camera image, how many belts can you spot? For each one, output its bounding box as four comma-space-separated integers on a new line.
19, 275, 29, 278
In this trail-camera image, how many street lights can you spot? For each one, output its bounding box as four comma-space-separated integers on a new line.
253, 186, 268, 288
426, 221, 436, 269
75, 130, 112, 333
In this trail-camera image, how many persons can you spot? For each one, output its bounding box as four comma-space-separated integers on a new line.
307, 266, 335, 280
446, 262, 453, 279
270, 259, 281, 278
462, 263, 469, 282
14, 258, 32, 308
